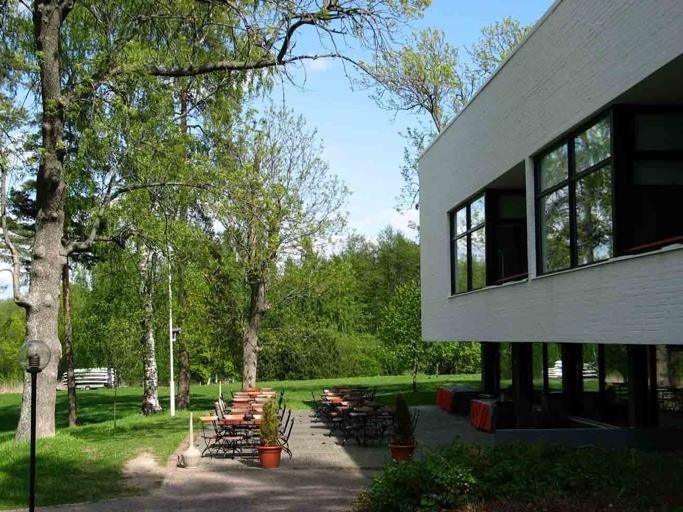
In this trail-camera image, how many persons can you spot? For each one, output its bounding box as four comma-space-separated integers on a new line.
386, 392, 416, 441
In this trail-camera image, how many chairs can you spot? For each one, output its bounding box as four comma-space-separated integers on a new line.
199, 388, 295, 460
311, 385, 421, 448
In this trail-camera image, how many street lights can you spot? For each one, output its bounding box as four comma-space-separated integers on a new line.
18, 340, 51, 512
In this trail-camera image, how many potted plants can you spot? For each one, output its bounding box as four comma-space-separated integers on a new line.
387, 396, 417, 460
257, 399, 283, 468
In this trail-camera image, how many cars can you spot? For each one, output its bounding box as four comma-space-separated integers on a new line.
61, 366, 116, 391
543, 360, 597, 380
